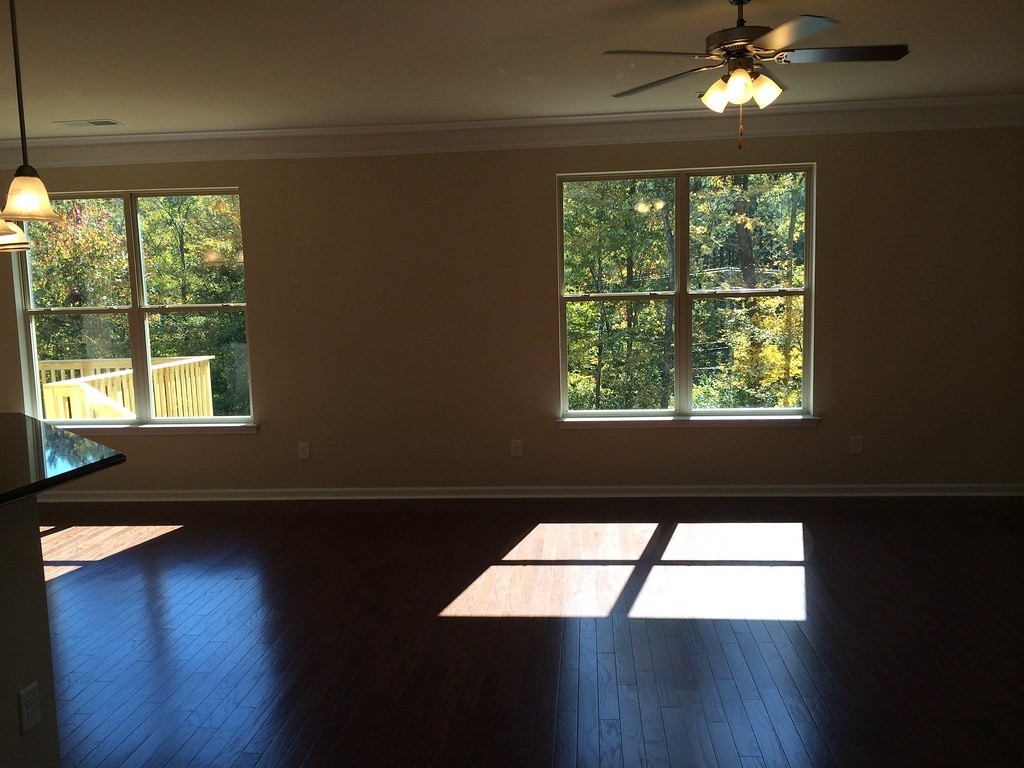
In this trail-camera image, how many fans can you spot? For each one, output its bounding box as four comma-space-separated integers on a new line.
602, 0, 910, 98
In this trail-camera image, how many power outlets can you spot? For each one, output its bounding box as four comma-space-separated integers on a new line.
18, 681, 43, 735
297, 442, 310, 461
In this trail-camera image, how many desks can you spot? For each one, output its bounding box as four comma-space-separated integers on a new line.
0, 412, 127, 768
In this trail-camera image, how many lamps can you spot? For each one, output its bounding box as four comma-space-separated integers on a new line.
699, 69, 784, 150
0, 0, 61, 254
